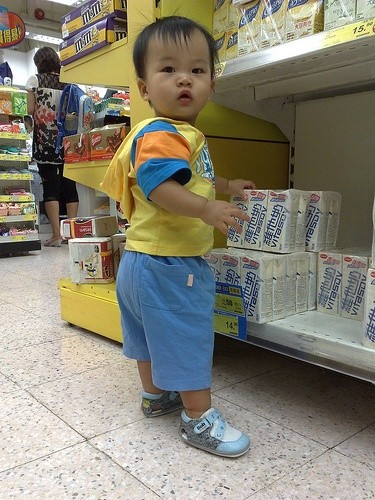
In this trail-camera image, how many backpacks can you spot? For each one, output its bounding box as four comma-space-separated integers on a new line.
55, 83, 94, 155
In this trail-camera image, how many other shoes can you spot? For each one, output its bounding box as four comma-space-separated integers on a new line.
178, 406, 250, 458
141, 388, 183, 417
44, 237, 62, 247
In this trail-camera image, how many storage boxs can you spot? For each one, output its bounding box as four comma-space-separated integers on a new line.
63, 123, 131, 164
57, 216, 128, 284
59, 0, 127, 67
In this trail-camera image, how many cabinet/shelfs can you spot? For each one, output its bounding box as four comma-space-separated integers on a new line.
0, 87, 42, 255
206, 0, 375, 386
58, 0, 289, 344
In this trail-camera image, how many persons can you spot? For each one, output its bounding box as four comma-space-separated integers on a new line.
23, 47, 79, 246
98, 16, 258, 458
